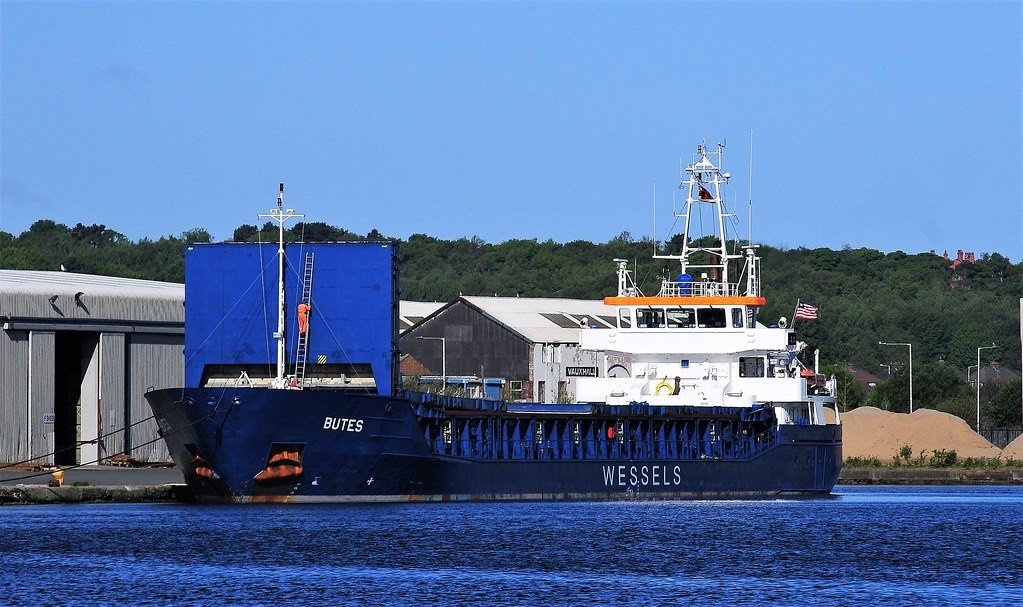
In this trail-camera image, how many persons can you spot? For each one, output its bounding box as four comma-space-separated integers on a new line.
53, 465, 64, 485
291, 381, 301, 390
297, 300, 310, 334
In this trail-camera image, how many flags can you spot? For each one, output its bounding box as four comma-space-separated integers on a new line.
794, 302, 818, 319
699, 184, 715, 203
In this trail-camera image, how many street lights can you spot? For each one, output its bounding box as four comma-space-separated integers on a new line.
968, 365, 978, 383
415, 335, 445, 395
978, 346, 999, 434
880, 364, 890, 376
880, 342, 913, 413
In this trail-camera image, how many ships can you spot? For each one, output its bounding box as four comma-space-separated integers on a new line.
144, 137, 845, 505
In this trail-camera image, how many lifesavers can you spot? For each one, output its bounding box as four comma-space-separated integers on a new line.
656, 381, 673, 395
